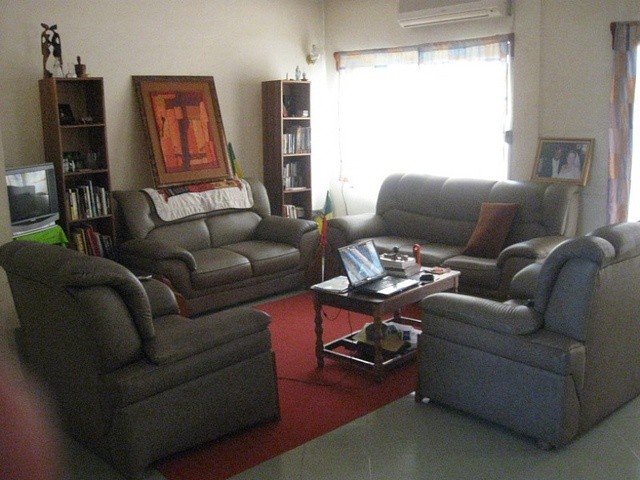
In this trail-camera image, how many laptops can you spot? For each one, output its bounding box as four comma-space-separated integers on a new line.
337, 239, 419, 298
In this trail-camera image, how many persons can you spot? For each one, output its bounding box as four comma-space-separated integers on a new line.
556, 150, 582, 180
539, 145, 564, 178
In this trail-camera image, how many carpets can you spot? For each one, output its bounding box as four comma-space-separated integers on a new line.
0, 291, 423, 479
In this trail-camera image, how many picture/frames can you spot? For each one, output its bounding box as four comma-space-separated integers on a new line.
531, 138, 595, 187
132, 75, 233, 190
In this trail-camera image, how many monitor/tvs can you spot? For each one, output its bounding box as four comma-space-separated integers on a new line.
5, 161, 61, 238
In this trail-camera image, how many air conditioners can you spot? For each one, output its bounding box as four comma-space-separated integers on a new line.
395, 1, 512, 30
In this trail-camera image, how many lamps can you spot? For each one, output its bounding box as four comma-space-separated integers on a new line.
306, 43, 320, 65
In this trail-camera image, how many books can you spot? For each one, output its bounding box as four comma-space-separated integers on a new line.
65, 169, 111, 220
380, 249, 417, 279
283, 121, 310, 155
282, 202, 312, 217
68, 224, 113, 258
285, 160, 311, 191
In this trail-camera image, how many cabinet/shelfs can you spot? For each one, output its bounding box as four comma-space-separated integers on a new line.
261, 80, 312, 222
38, 78, 118, 262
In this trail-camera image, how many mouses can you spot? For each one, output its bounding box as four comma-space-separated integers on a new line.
419, 273, 434, 283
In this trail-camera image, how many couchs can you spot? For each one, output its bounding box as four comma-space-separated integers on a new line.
325, 174, 584, 299
0, 239, 280, 480
112, 178, 322, 318
413, 219, 640, 452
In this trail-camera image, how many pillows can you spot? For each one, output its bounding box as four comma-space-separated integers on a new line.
462, 203, 520, 259
